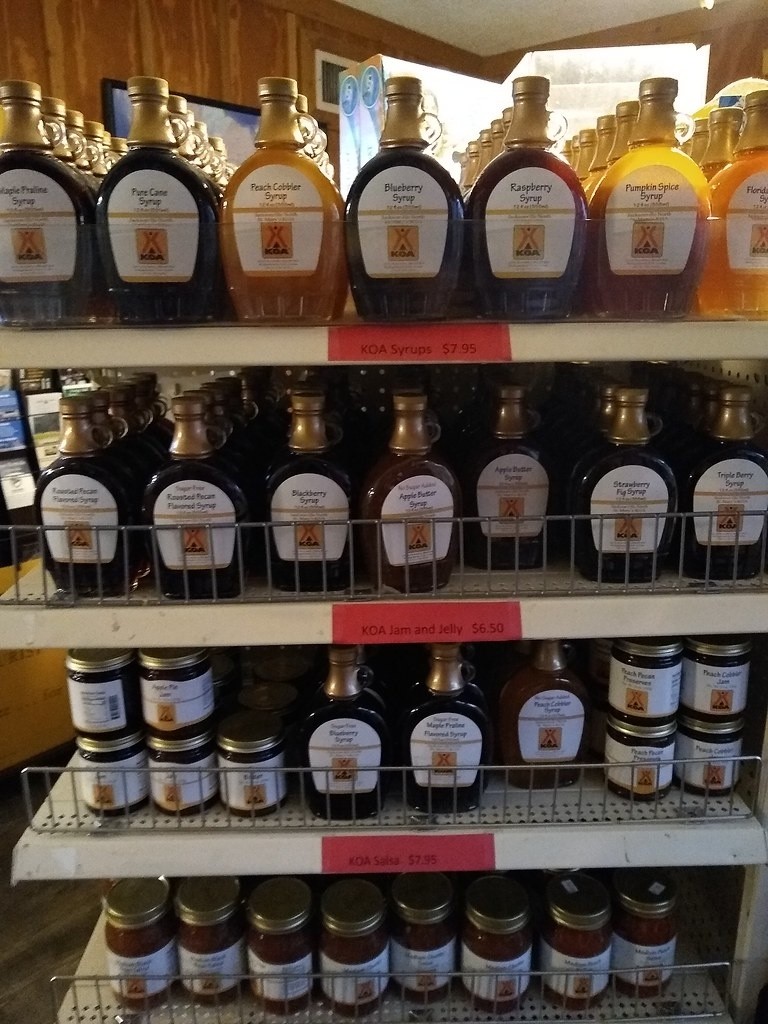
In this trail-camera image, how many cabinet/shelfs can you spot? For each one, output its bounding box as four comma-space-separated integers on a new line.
1, 77, 768, 1022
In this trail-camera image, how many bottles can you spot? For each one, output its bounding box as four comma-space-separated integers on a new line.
36, 372, 768, 602
0, 76, 768, 324
301, 639, 592, 822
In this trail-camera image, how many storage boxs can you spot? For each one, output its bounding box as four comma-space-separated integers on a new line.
338, 55, 502, 202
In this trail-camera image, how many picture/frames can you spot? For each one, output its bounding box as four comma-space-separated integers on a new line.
100, 77, 329, 165
